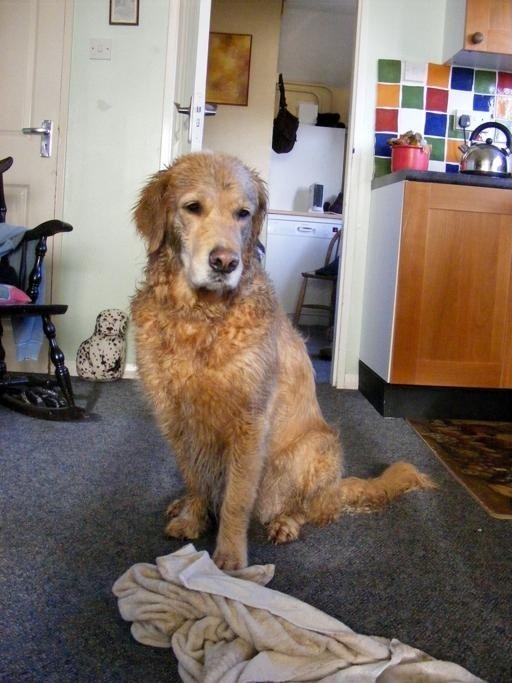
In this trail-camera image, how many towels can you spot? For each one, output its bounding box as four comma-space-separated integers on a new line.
108, 540, 482, 683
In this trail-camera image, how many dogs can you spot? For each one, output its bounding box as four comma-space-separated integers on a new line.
123, 148, 442, 573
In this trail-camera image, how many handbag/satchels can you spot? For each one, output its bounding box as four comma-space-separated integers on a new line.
272, 107, 299, 153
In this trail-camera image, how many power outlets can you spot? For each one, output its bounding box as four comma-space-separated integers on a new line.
453, 111, 492, 135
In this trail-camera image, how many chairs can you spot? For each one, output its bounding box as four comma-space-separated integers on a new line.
291, 228, 342, 345
1, 157, 87, 425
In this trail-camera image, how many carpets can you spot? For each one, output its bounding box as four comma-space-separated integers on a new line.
404, 414, 511, 519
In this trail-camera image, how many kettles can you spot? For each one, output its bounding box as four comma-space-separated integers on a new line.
453, 115, 512, 179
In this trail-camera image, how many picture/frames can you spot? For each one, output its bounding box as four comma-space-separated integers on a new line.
206, 33, 252, 106
108, 1, 140, 27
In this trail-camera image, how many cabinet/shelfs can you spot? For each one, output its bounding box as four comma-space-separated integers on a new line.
265, 214, 344, 326
443, 0, 511, 76
358, 182, 511, 423
